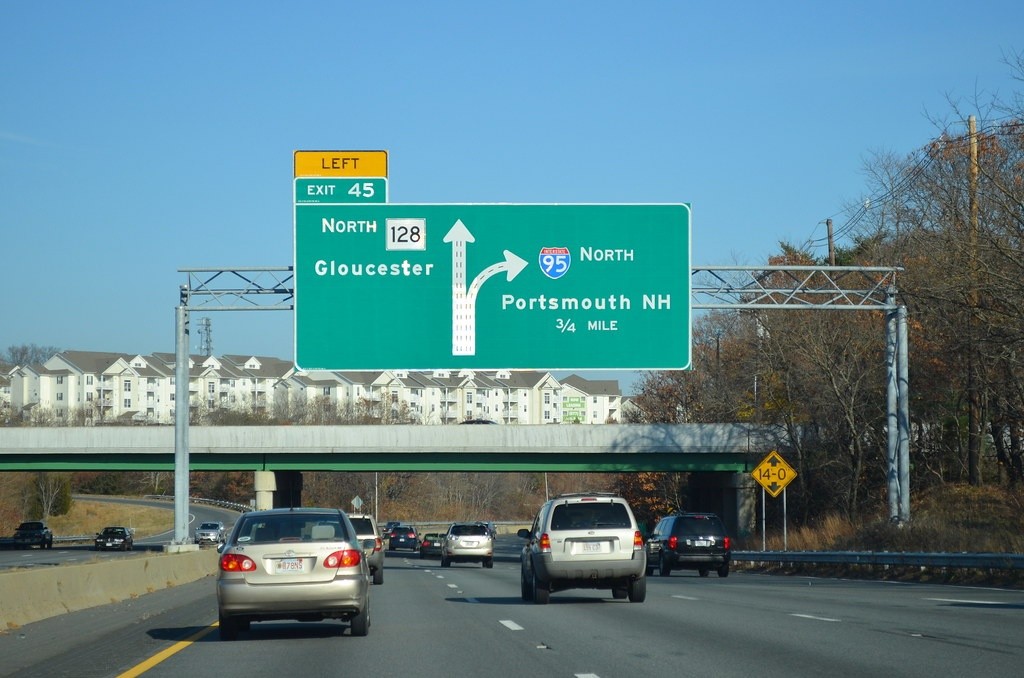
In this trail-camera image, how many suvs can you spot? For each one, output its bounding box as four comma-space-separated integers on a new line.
644, 512, 731, 578
517, 491, 648, 605
195, 520, 227, 545
441, 522, 496, 568
389, 525, 422, 552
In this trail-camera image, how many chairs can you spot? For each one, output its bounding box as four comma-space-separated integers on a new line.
311, 525, 335, 540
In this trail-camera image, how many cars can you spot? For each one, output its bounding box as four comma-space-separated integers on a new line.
420, 533, 448, 559
377, 520, 400, 538
217, 507, 376, 641
12, 521, 53, 549
94, 527, 133, 552
345, 513, 385, 585
